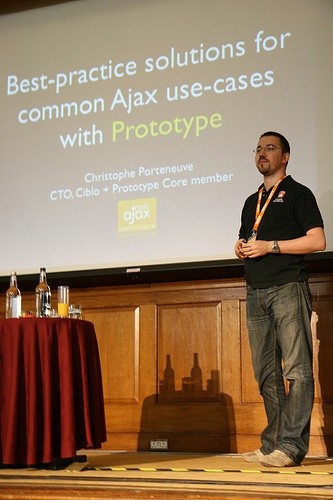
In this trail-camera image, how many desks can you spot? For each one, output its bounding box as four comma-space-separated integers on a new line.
0, 316, 106, 465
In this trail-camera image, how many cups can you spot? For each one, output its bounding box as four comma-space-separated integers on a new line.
39, 292, 52, 317
57, 285, 69, 318
69, 303, 83, 319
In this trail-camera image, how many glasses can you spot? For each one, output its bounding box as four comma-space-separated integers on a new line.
253, 146, 281, 153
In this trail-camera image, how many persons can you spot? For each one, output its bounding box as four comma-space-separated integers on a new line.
234, 131, 326, 467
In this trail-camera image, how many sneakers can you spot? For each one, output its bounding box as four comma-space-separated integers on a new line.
259, 449, 301, 466
242, 448, 265, 463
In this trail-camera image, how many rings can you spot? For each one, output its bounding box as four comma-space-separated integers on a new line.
249, 248, 251, 251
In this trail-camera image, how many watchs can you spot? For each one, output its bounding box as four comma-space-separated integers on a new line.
272, 241, 280, 253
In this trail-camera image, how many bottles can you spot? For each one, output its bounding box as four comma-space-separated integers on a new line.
35, 267, 51, 317
5, 271, 22, 318
